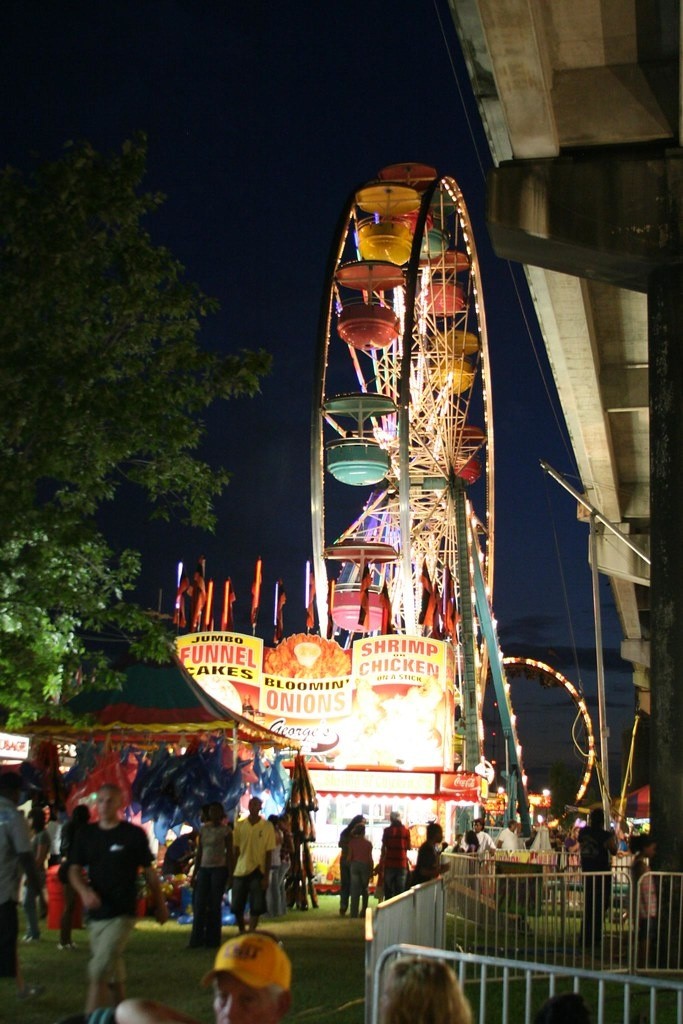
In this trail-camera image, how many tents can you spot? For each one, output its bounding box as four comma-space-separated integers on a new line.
0, 636, 300, 771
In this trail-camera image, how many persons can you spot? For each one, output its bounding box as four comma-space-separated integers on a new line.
0, 761, 658, 1024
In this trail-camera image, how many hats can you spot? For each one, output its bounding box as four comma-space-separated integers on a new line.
201, 933, 292, 992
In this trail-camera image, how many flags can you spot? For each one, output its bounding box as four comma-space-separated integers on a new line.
169, 556, 462, 645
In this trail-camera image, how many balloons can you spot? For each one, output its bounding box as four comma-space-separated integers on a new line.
55, 735, 247, 830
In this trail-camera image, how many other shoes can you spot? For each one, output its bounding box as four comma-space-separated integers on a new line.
26, 936, 38, 942
57, 943, 70, 951
21, 935, 28, 942
203, 941, 220, 950
70, 942, 78, 950
186, 940, 203, 949
